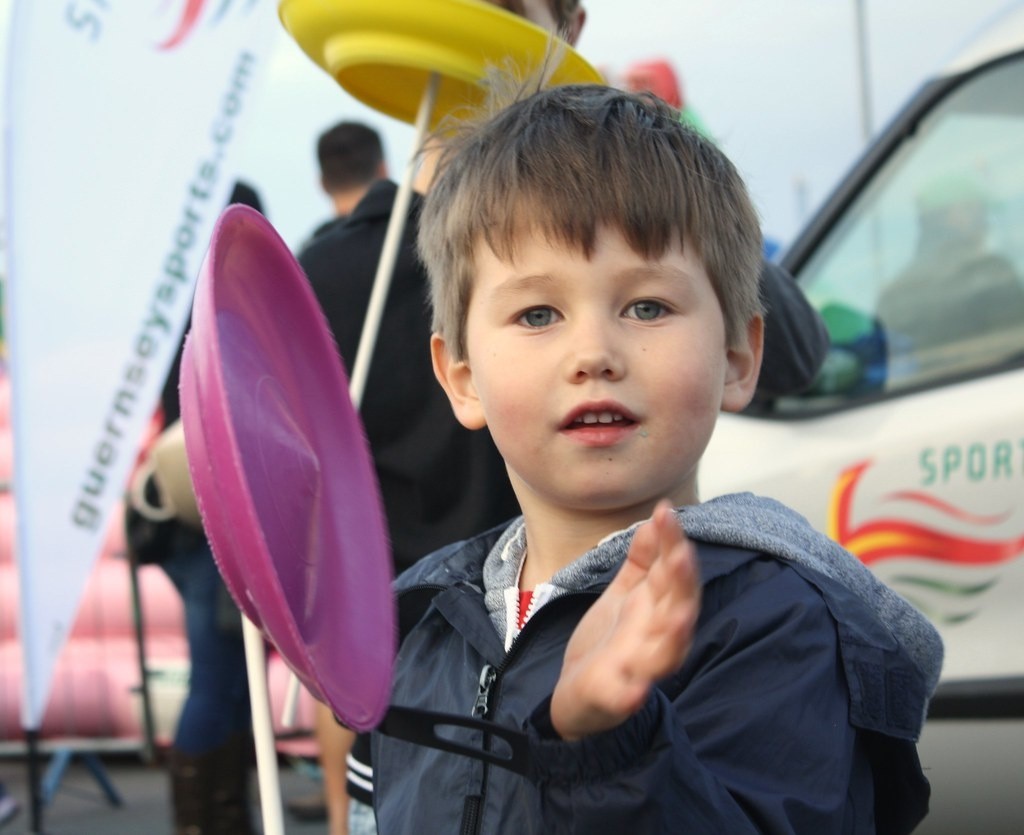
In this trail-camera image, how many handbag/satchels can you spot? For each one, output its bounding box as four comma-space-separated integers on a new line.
127, 480, 173, 564
753, 260, 830, 393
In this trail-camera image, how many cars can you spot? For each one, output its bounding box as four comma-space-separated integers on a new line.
692, 0, 1024, 718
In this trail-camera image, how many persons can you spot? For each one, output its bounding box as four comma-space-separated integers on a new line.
371, 87, 944, 835
127, 0, 830, 835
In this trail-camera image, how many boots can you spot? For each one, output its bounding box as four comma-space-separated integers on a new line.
171, 728, 255, 835
